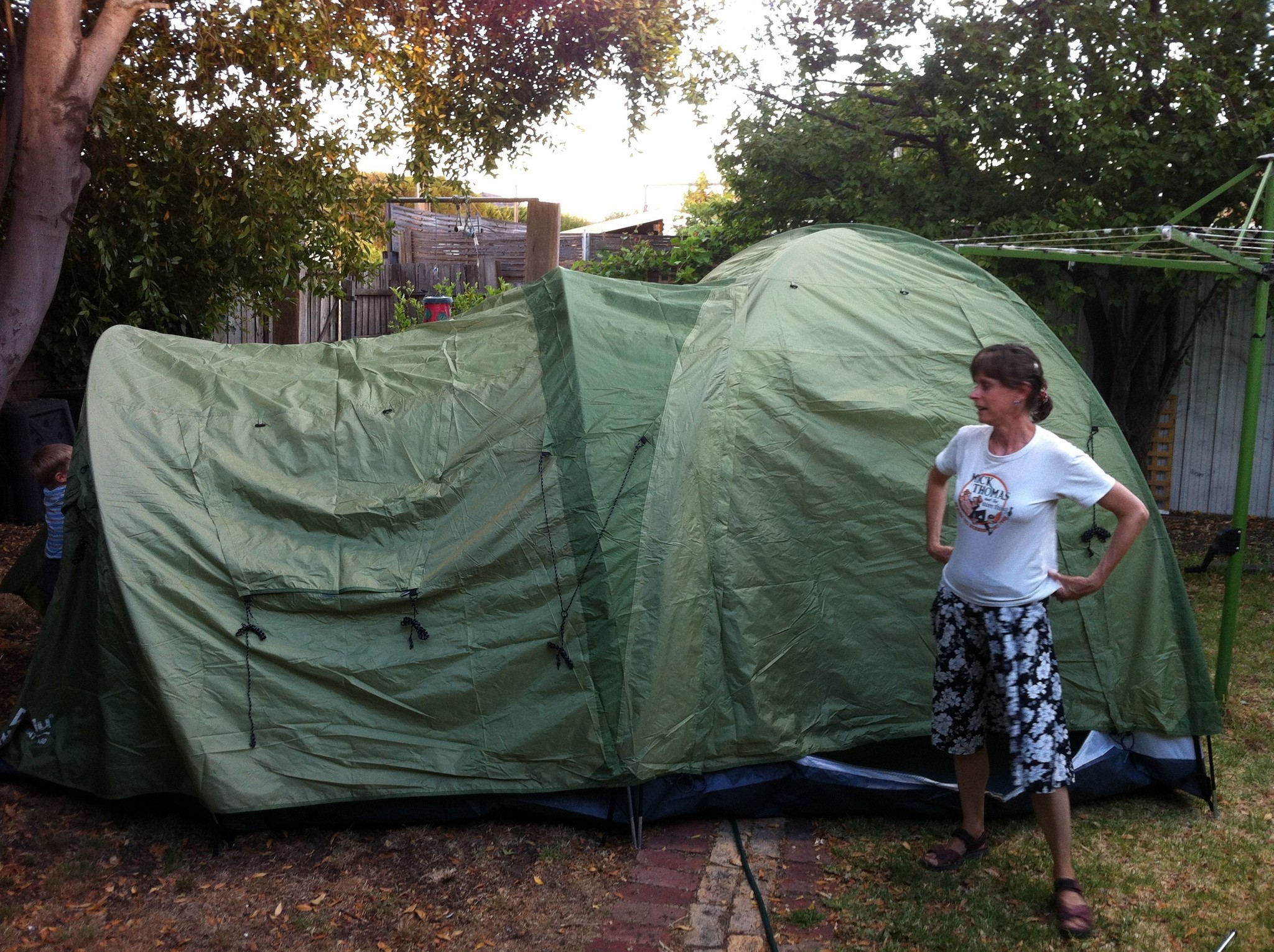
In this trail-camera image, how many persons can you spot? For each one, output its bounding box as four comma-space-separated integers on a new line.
925, 344, 1149, 938
28, 443, 74, 604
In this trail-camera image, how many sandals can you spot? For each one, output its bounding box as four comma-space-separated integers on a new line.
921, 827, 989, 871
1054, 878, 1092, 941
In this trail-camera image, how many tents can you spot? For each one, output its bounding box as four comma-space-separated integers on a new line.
1, 224, 1228, 850
